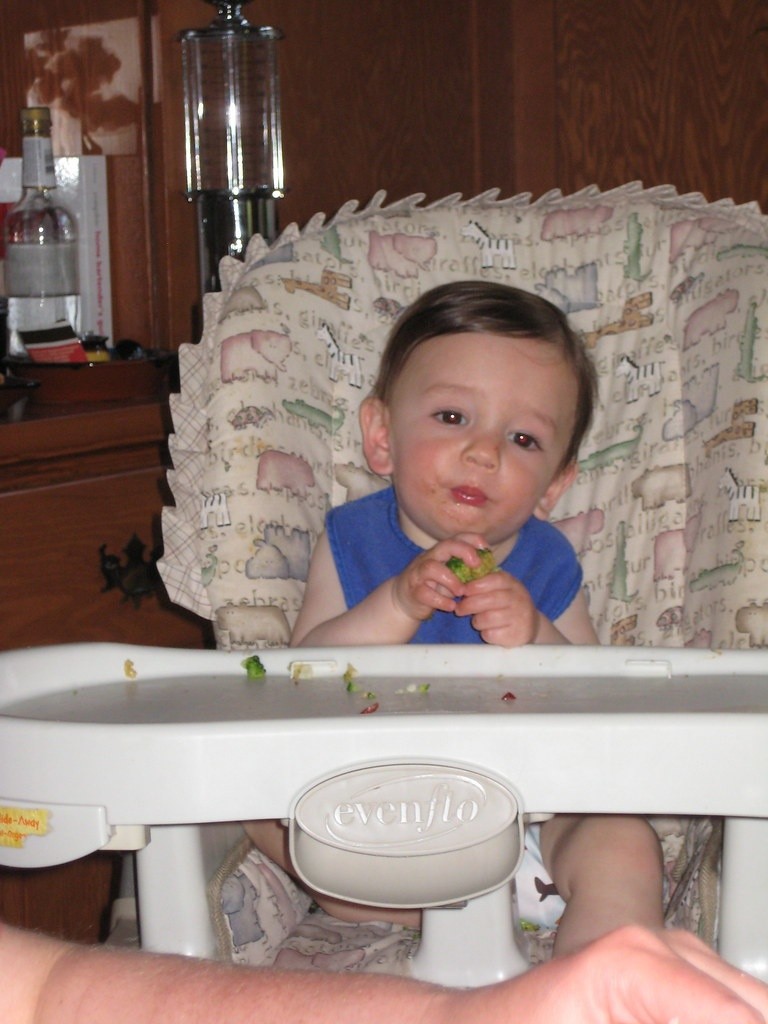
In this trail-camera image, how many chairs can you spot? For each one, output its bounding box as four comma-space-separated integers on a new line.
105, 180, 768, 976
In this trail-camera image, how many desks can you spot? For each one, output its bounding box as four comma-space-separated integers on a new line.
0, 360, 216, 650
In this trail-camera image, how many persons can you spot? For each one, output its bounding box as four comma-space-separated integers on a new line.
0, 914, 768, 1023
233, 278, 666, 963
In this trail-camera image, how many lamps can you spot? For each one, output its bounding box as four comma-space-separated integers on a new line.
176, 0, 303, 344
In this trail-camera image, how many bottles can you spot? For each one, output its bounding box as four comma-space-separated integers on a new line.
4, 106, 81, 357
